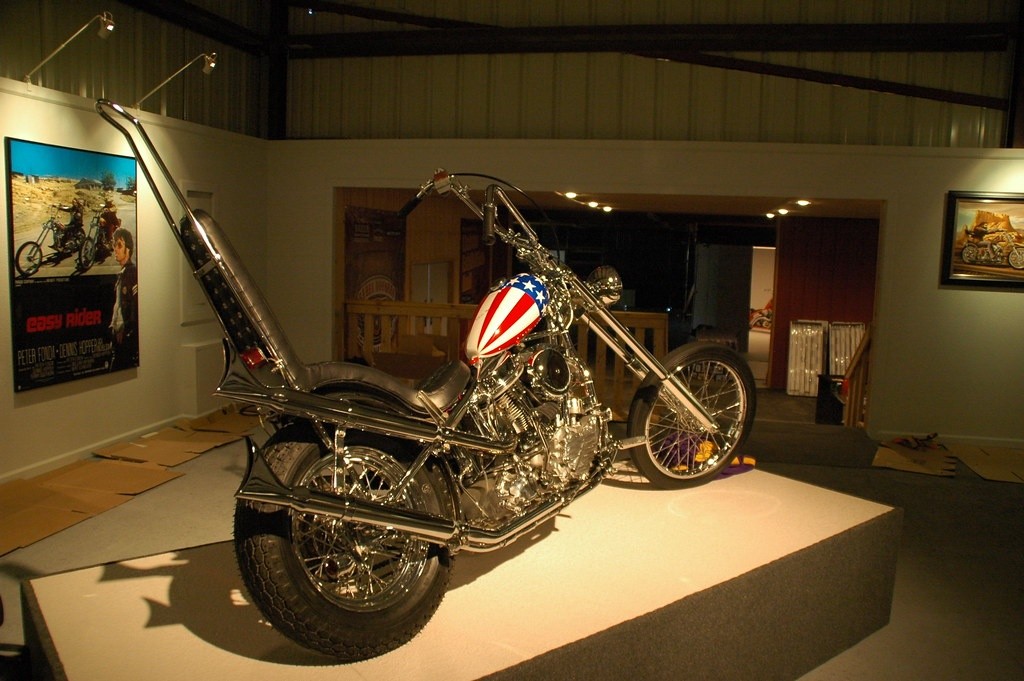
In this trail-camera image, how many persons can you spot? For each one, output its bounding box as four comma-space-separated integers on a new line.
47, 196, 118, 258
106, 228, 138, 373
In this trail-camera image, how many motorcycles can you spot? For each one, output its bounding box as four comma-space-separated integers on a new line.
79, 202, 122, 271
960, 229, 1023, 271
86, 95, 761, 663
13, 203, 89, 276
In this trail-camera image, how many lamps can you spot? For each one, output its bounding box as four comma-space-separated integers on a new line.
130, 52, 218, 110
21, 11, 115, 84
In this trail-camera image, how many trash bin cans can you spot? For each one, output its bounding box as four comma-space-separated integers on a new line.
815, 373, 845, 424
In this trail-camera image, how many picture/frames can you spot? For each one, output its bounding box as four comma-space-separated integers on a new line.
938, 189, 1024, 290
